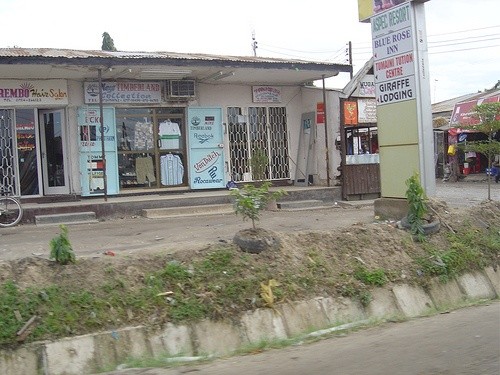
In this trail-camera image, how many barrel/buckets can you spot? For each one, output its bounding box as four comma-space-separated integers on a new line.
448, 144, 456, 156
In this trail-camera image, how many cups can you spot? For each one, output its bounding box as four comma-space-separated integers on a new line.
463, 168, 469, 175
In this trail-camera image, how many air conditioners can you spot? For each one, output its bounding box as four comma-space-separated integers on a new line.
164, 79, 197, 100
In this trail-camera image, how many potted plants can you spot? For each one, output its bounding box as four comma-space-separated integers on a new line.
401, 172, 441, 237
228, 181, 287, 253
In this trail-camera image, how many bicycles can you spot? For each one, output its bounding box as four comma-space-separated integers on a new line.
0, 190, 24, 227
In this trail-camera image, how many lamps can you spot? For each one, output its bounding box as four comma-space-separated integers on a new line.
101, 68, 112, 78
215, 72, 236, 79
139, 67, 191, 74
113, 69, 131, 80
202, 71, 222, 80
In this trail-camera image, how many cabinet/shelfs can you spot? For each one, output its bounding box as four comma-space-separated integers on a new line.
121, 175, 145, 188
250, 146, 269, 188
